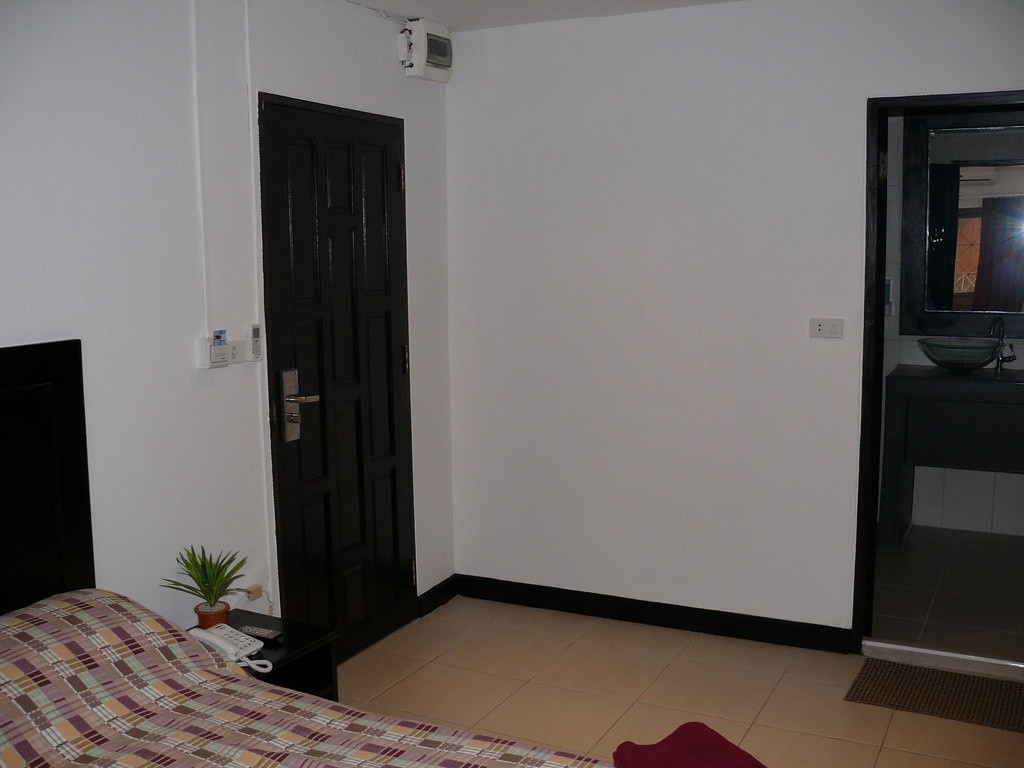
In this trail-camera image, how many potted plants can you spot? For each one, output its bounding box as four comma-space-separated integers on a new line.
157, 544, 252, 629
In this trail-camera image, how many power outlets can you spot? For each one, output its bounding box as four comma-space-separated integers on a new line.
809, 318, 843, 338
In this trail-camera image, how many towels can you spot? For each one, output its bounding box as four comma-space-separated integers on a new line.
613, 722, 766, 768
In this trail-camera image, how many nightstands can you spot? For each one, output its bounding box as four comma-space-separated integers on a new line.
187, 607, 342, 705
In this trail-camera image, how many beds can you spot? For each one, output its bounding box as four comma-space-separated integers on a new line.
1, 338, 623, 768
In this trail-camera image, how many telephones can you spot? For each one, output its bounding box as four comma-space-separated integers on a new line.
187, 622, 273, 673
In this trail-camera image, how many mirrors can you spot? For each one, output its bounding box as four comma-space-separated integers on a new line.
923, 127, 1023, 314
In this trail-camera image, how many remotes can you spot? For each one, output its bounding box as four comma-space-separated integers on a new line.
238, 625, 282, 639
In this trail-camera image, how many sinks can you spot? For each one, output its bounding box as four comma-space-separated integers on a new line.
917, 338, 1006, 372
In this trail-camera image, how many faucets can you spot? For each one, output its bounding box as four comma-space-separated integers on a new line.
988, 316, 1016, 374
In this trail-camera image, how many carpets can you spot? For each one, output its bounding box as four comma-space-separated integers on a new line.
843, 659, 1024, 734
